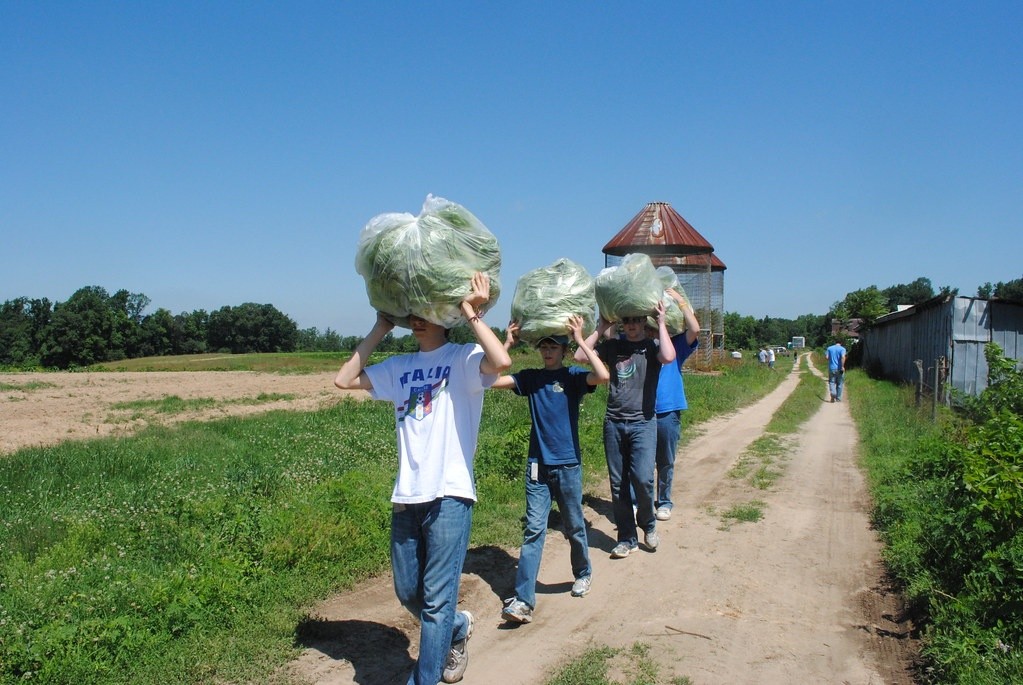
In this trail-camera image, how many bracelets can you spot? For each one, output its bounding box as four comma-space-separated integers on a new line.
679, 302, 687, 309
467, 313, 481, 324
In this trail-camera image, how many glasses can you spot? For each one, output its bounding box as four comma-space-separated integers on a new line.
622, 318, 643, 324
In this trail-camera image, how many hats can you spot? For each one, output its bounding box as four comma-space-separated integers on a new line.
536, 336, 568, 345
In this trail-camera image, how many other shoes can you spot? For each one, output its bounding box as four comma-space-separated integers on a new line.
831, 396, 840, 403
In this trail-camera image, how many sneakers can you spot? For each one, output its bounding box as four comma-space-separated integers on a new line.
632, 504, 638, 527
656, 507, 671, 520
571, 573, 592, 597
442, 610, 475, 683
611, 543, 639, 558
501, 595, 532, 623
644, 531, 658, 550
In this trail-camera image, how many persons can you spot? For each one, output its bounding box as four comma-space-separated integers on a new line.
825, 337, 846, 403
572, 298, 677, 561
490, 313, 611, 627
603, 287, 703, 529
330, 272, 516, 685
757, 347, 799, 372
